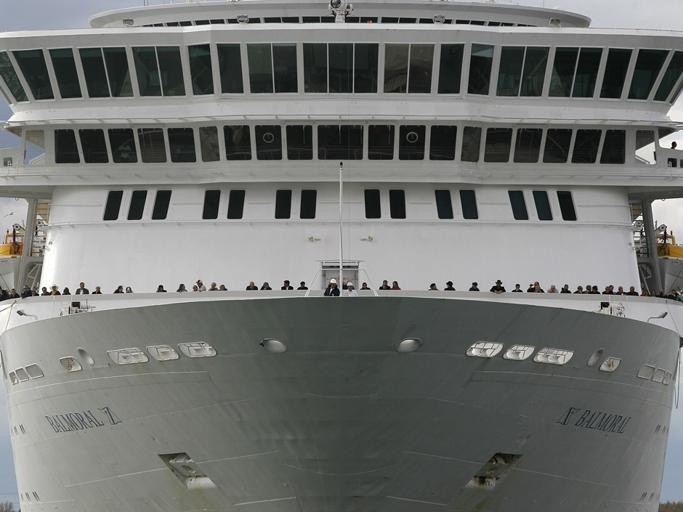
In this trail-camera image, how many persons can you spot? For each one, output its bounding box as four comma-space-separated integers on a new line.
261, 283, 271, 290
298, 281, 308, 289
156, 286, 166, 292
511, 281, 683, 303
469, 282, 479, 292
246, 282, 258, 290
281, 280, 293, 290
443, 281, 456, 291
428, 282, 437, 290
490, 280, 506, 294
0, 282, 133, 301
176, 280, 228, 293
670, 141, 678, 149
323, 278, 399, 297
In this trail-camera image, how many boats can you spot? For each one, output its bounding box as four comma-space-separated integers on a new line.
0, 229, 42, 299
636, 228, 682, 297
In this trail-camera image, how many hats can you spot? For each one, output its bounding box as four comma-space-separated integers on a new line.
330, 278, 336, 283
496, 280, 502, 284
347, 282, 353, 286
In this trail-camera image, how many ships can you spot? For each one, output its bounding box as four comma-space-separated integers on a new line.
0, 0, 682, 512
